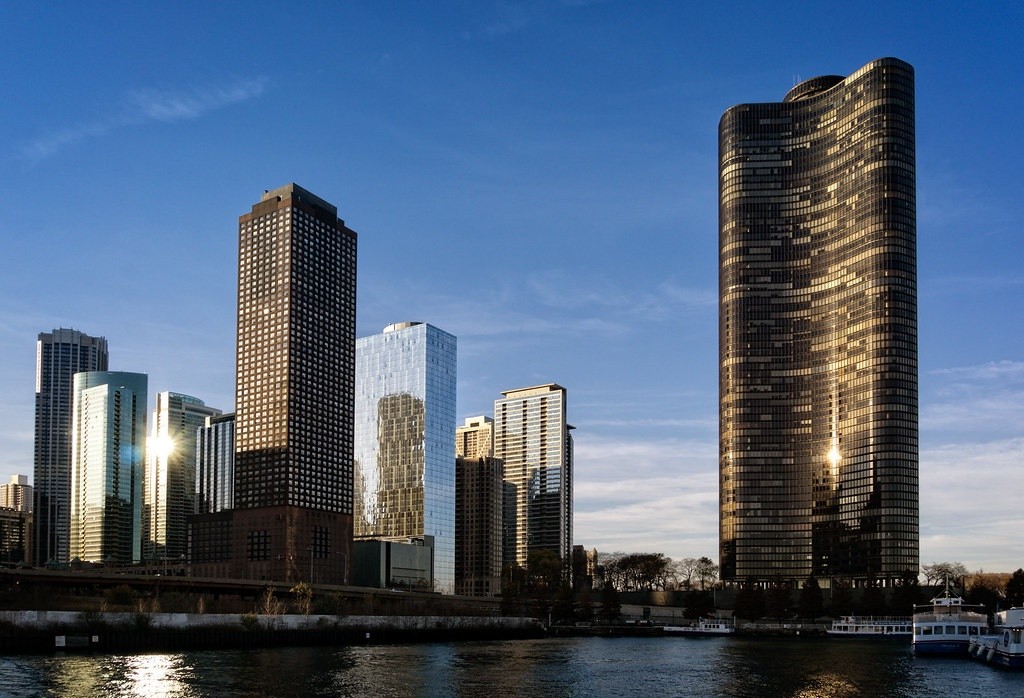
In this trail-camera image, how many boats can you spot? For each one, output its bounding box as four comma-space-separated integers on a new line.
822, 614, 912, 638
968, 604, 1024, 670
663, 616, 735, 634
911, 570, 989, 652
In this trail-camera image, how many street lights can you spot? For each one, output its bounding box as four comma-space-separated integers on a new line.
307, 547, 312, 583
337, 551, 347, 584
